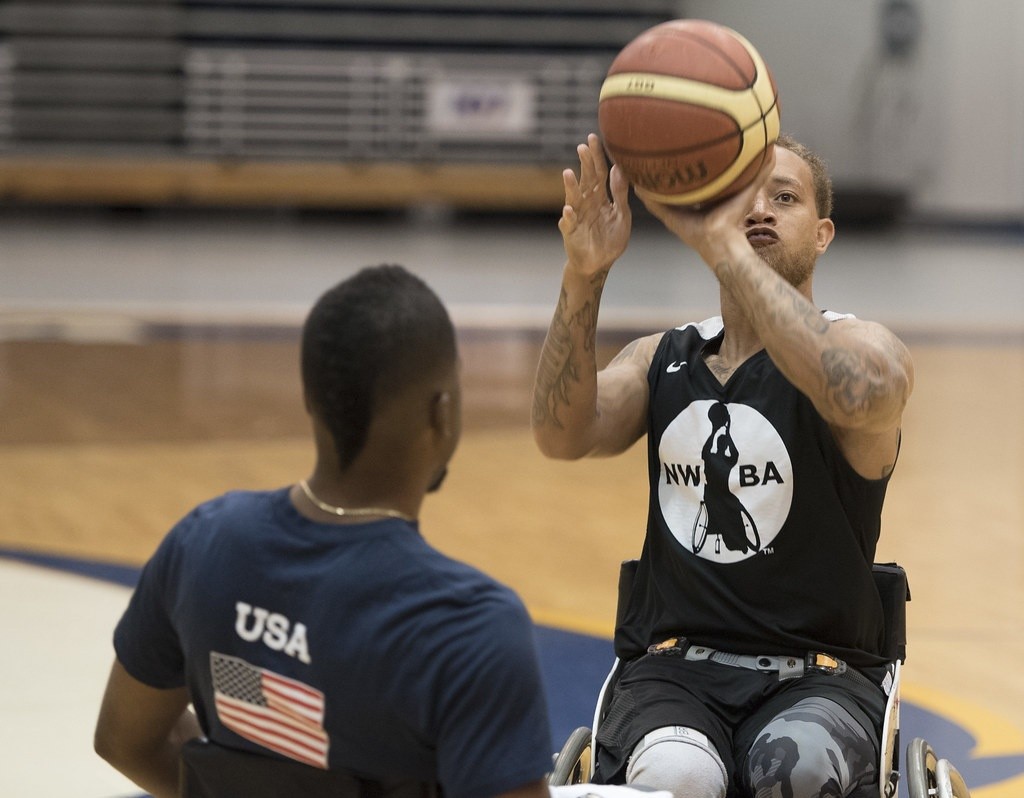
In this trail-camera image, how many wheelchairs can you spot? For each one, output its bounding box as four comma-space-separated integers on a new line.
548, 562, 972, 798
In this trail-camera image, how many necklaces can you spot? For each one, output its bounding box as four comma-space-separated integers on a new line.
296, 479, 406, 520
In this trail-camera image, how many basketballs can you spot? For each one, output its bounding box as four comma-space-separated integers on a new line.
597, 17, 783, 211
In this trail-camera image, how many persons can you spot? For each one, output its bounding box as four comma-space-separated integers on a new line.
532, 131, 914, 798
95, 263, 555, 798
